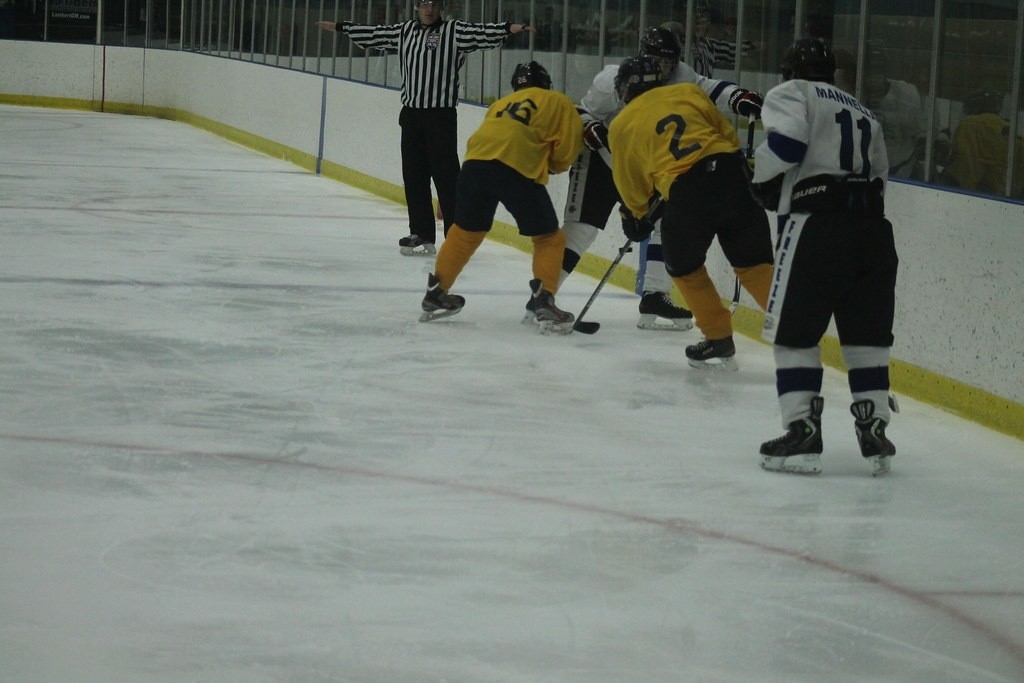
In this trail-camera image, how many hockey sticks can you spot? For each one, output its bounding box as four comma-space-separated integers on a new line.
570, 191, 666, 335
722, 112, 756, 316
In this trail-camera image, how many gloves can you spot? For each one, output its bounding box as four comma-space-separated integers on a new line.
618, 207, 655, 243
728, 88, 764, 119
583, 120, 610, 152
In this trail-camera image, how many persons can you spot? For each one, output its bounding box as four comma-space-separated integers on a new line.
523, 27, 765, 331
750, 38, 899, 478
418, 61, 583, 336
677, 5, 1024, 203
315, 0, 537, 257
606, 53, 775, 373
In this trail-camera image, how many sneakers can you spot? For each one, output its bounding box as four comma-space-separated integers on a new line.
636, 291, 693, 331
850, 399, 895, 477
398, 234, 437, 256
758, 396, 825, 473
520, 278, 575, 336
685, 335, 738, 371
417, 272, 467, 322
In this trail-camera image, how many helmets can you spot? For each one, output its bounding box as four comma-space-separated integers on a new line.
413, 0, 447, 19
849, 45, 887, 76
776, 37, 837, 84
961, 76, 1004, 118
680, 3, 711, 37
613, 54, 668, 107
639, 25, 681, 81
510, 61, 554, 92
